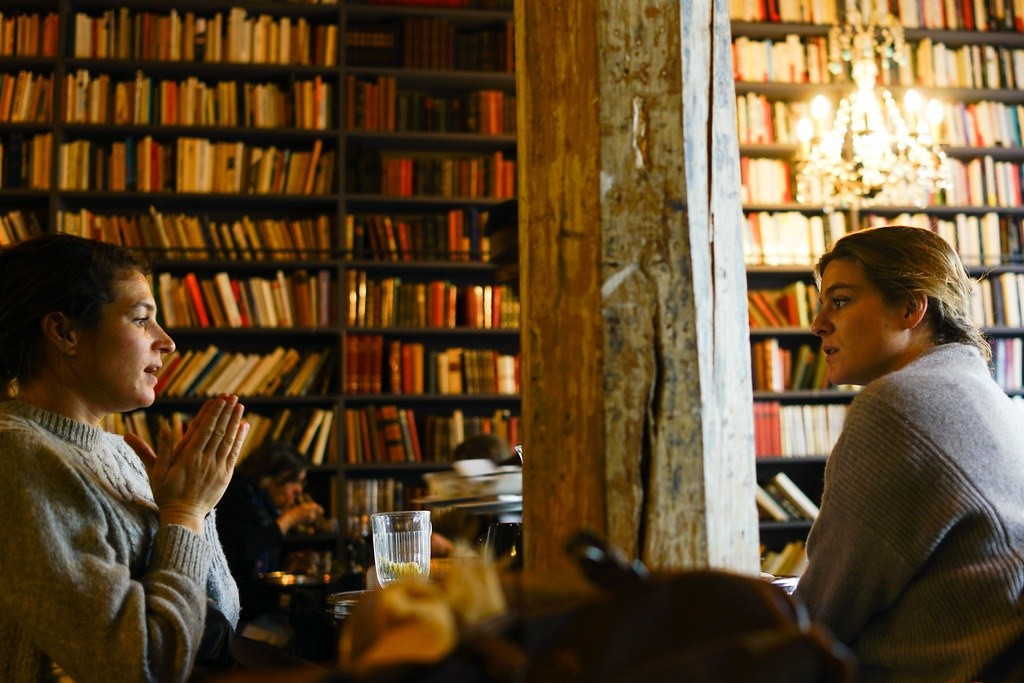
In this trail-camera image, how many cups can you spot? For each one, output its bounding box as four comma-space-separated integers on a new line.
370, 511, 433, 589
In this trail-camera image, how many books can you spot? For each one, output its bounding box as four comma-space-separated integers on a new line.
0, 1, 1024, 542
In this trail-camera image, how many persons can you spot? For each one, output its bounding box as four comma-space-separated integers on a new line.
429, 434, 518, 560
219, 441, 329, 624
786, 227, 1024, 683
0, 233, 250, 683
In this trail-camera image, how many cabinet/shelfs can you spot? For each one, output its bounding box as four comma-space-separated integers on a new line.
0, 0, 522, 543
729, 17, 1024, 549
